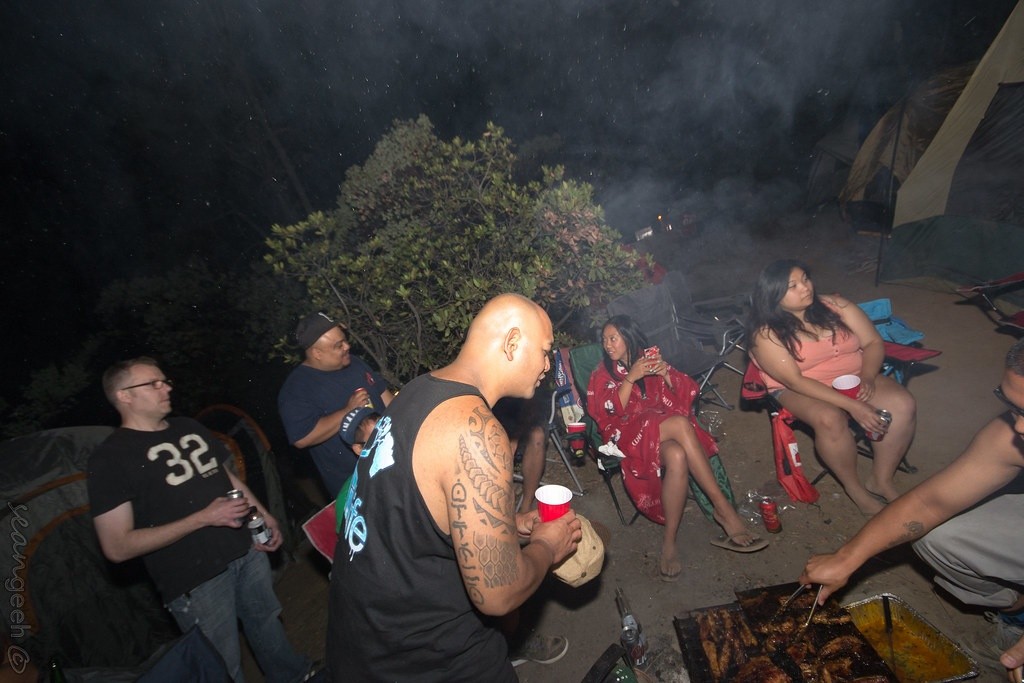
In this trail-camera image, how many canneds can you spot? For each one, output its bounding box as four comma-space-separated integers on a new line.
866, 410, 892, 442
226, 489, 246, 523
758, 497, 782, 533
355, 387, 373, 409
620, 626, 648, 670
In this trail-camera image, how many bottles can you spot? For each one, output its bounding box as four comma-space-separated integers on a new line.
614, 587, 649, 652
246, 506, 273, 544
761, 496, 783, 533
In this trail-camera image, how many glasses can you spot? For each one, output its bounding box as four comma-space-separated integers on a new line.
121, 378, 174, 389
993, 384, 1024, 418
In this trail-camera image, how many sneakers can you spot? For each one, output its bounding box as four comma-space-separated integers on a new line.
982, 605, 1024, 636
507, 623, 568, 667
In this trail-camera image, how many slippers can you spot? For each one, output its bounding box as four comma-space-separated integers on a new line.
657, 547, 683, 583
711, 534, 772, 554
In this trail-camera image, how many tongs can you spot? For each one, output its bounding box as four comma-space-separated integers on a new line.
769, 584, 823, 644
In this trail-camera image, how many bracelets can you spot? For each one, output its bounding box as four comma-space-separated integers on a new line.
624, 377, 634, 385
662, 370, 668, 376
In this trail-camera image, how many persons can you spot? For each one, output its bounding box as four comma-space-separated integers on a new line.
334, 407, 380, 536
483, 599, 569, 666
492, 387, 549, 513
798, 338, 1024, 682
279, 313, 395, 498
87, 355, 316, 683
745, 257, 916, 517
587, 313, 769, 580
327, 292, 582, 683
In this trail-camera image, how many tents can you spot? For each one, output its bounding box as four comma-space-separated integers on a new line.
808, 0, 1024, 329
0, 426, 234, 683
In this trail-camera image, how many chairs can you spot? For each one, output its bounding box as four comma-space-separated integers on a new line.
515, 270, 942, 526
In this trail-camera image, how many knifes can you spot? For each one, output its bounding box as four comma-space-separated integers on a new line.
882, 596, 896, 670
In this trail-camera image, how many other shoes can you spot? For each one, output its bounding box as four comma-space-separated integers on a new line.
298, 657, 325, 683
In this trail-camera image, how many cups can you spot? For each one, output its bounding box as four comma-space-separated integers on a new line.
568, 423, 586, 457
832, 375, 861, 401
535, 483, 572, 524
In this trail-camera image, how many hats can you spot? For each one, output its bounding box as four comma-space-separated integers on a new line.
295, 311, 340, 351
549, 514, 604, 588
339, 407, 379, 445
